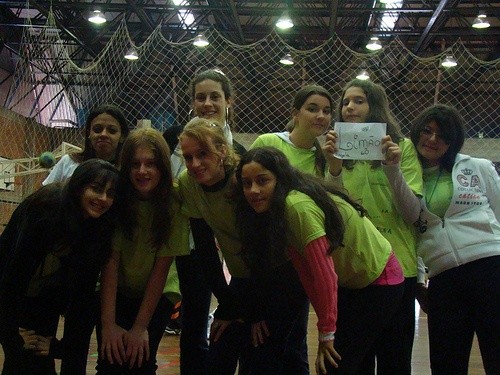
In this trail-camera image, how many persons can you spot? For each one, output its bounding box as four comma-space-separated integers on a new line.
0, 70, 425, 375
410, 104, 500, 375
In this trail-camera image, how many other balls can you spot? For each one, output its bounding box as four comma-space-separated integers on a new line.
39, 151, 56, 169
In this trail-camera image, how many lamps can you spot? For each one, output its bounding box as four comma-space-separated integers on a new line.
276, 10, 294, 29
193, 31, 210, 47
366, 36, 383, 50
279, 54, 294, 65
88, 6, 106, 23
472, 14, 490, 28
356, 70, 369, 80
441, 52, 457, 66
124, 48, 139, 60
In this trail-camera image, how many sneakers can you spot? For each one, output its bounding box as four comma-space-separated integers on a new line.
165, 326, 181, 335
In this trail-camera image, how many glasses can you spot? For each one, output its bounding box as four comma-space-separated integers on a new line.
422, 128, 443, 138
